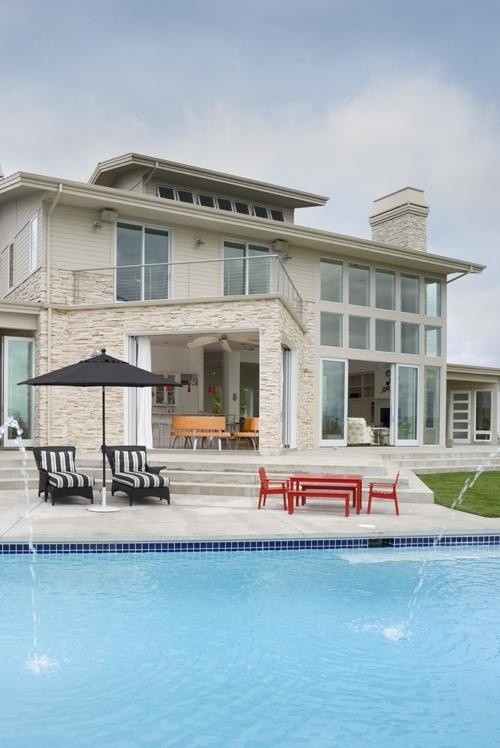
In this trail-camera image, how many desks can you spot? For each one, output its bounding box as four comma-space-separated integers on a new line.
242, 417, 259, 450
372, 427, 388, 446
171, 415, 226, 450
289, 476, 363, 514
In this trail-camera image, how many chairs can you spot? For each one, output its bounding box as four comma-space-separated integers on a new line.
257, 466, 290, 510
346, 416, 371, 445
383, 422, 411, 446
105, 445, 170, 506
32, 447, 94, 506
367, 470, 400, 516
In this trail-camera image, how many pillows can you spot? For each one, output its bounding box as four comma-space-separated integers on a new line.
40, 449, 74, 472
114, 449, 146, 475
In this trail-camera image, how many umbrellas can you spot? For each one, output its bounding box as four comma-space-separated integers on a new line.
16, 346, 185, 507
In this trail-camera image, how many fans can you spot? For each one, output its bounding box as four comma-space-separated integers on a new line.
187, 334, 259, 352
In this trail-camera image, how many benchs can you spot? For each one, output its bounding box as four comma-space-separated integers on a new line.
287, 491, 350, 517
171, 431, 231, 449
232, 432, 259, 450
300, 483, 357, 507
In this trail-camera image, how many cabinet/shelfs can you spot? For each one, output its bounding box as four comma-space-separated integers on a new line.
151, 372, 176, 407
348, 371, 375, 400
